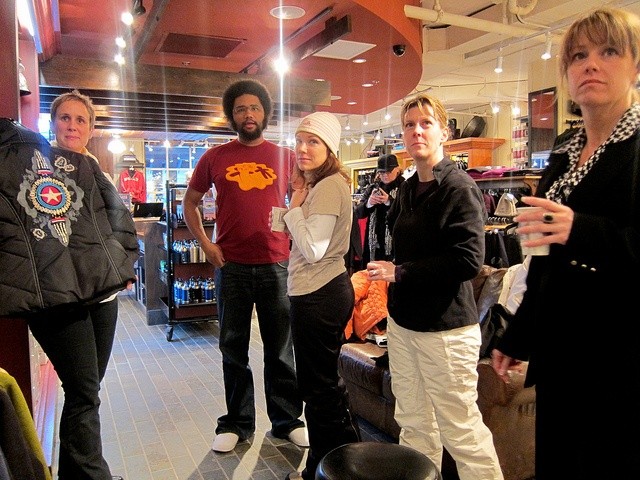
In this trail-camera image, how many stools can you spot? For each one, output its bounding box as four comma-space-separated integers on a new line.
315, 443, 439, 478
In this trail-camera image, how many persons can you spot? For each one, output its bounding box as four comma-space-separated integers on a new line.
182, 78, 311, 453
267, 112, 363, 480
354, 154, 407, 270
365, 93, 504, 480
491, 5, 640, 480
25, 89, 140, 480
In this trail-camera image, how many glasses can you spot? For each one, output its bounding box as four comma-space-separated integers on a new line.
232, 104, 263, 114
378, 171, 392, 174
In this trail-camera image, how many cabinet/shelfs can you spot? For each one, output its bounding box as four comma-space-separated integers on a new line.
344, 156, 380, 229
473, 175, 542, 207
394, 136, 505, 181
512, 116, 528, 168
165, 182, 220, 342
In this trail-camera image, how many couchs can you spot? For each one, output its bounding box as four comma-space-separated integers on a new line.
337, 262, 537, 478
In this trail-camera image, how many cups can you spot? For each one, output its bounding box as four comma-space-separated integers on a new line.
269, 204, 290, 234
515, 204, 553, 258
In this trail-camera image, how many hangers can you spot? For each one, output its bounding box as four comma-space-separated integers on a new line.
127, 165, 136, 174
484, 215, 518, 236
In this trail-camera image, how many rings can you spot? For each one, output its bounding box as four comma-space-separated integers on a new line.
542, 210, 554, 224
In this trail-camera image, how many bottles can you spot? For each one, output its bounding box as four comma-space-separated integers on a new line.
173, 238, 215, 264
174, 273, 216, 304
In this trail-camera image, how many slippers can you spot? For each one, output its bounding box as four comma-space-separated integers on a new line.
286, 472, 304, 480
213, 433, 239, 452
288, 427, 310, 446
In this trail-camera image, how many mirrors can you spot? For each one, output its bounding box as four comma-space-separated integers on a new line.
527, 85, 559, 170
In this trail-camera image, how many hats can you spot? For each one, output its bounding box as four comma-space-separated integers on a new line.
295, 111, 341, 158
377, 154, 399, 171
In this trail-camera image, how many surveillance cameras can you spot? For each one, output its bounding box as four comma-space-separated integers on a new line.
392, 44, 407, 57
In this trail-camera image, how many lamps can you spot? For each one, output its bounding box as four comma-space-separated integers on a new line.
491, 45, 508, 74
542, 37, 555, 60
114, 28, 137, 49
120, 0, 146, 25
